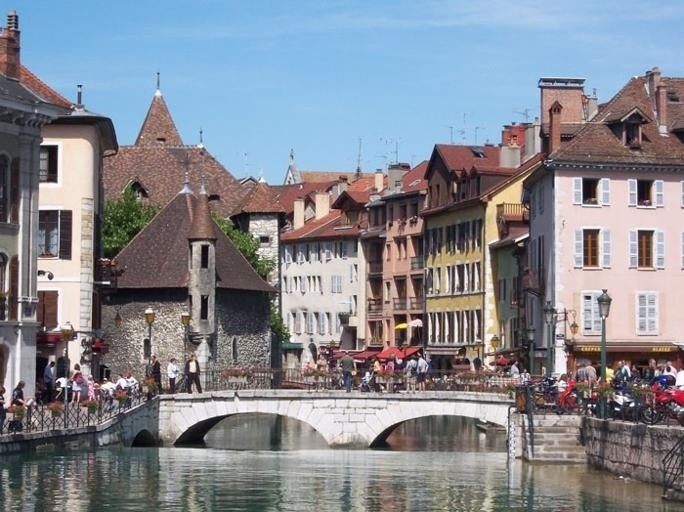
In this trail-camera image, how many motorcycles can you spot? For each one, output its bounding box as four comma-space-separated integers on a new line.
523, 371, 684, 426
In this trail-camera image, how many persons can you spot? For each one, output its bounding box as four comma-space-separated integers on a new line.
87, 373, 139, 411
511, 360, 530, 382
542, 363, 546, 376
36, 361, 82, 405
142, 353, 203, 401
558, 358, 683, 418
13, 381, 28, 431
305, 353, 429, 393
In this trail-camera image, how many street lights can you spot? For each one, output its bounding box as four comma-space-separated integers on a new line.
143, 304, 155, 376
526, 323, 536, 376
595, 287, 612, 414
179, 305, 190, 365
490, 334, 500, 372
59, 320, 73, 428
474, 338, 482, 359
543, 299, 556, 376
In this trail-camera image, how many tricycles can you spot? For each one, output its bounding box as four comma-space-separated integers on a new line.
357, 369, 402, 392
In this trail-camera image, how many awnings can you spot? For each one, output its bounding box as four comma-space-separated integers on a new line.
330, 350, 418, 360
